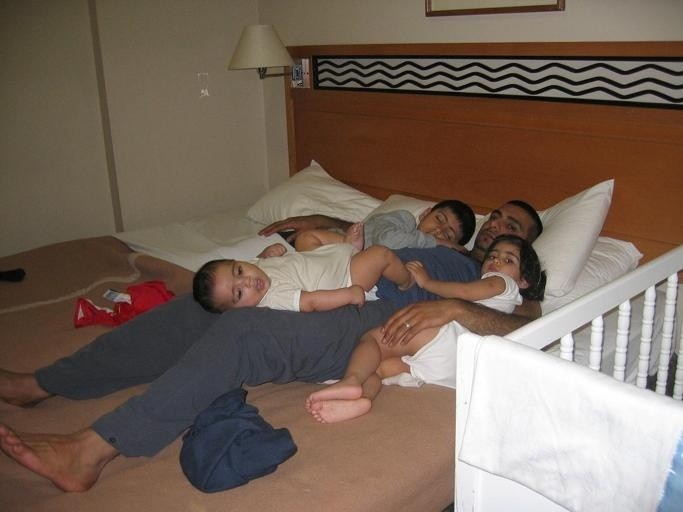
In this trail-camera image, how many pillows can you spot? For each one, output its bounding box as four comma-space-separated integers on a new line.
362, 194, 484, 243
473, 179, 615, 293
245, 159, 384, 233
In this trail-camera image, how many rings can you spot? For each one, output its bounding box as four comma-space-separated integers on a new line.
404, 322, 411, 329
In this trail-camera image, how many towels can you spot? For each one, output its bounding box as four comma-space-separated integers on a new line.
457, 333, 683, 512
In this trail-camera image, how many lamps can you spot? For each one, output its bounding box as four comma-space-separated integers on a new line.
225, 25, 294, 82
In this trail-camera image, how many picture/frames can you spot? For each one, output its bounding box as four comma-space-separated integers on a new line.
422, 1, 564, 16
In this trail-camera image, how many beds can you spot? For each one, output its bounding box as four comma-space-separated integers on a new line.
0, 43, 682, 512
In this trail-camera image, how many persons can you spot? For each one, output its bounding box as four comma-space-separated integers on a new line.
362, 199, 476, 255
192, 221, 416, 313
0, 199, 544, 495
302, 235, 547, 425
